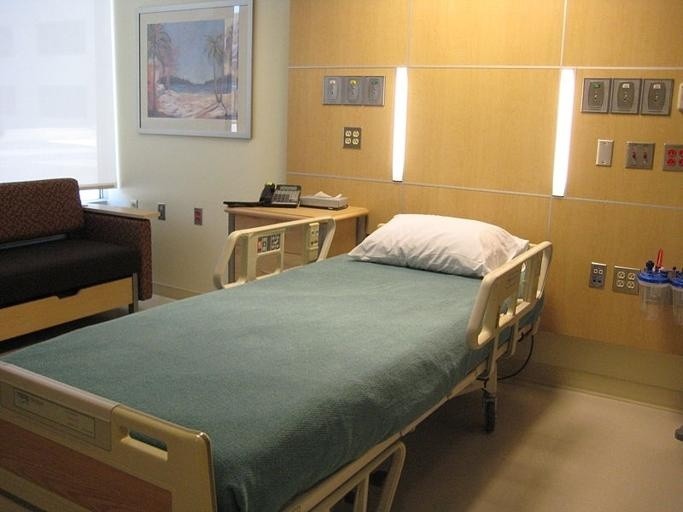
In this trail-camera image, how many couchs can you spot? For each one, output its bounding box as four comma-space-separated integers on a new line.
0, 177, 155, 345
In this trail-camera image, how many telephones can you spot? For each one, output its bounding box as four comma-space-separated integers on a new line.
259, 183, 301, 208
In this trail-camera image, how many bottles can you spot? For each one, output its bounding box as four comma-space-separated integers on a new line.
670, 273, 683, 325
638, 272, 670, 321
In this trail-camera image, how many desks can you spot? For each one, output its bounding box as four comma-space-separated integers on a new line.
223, 202, 368, 283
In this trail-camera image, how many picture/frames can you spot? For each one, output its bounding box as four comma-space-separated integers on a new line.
136, 0, 254, 141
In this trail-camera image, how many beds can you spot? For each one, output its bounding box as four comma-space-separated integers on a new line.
0, 211, 554, 511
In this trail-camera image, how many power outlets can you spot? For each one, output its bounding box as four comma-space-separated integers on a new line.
343, 125, 361, 152
587, 261, 640, 296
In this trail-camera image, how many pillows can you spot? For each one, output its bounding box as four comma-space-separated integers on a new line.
346, 213, 530, 279
